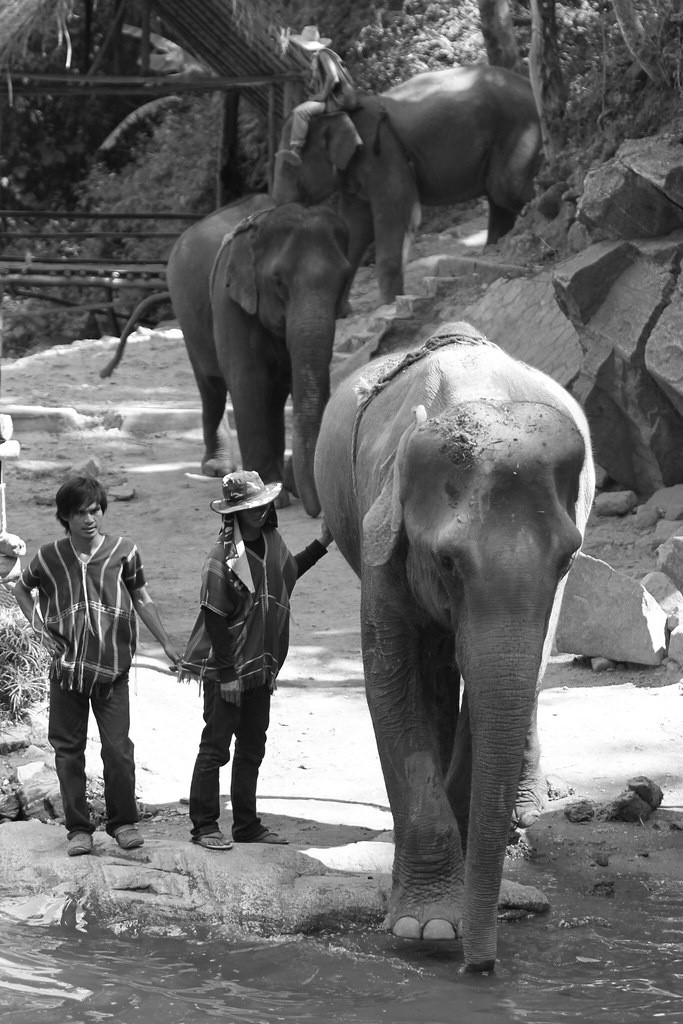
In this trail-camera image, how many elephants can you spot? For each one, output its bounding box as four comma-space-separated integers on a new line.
267, 61, 547, 312
311, 315, 602, 973
98, 189, 371, 519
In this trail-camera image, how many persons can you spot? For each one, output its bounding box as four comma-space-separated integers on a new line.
177, 470, 334, 849
14, 476, 179, 856
274, 26, 354, 167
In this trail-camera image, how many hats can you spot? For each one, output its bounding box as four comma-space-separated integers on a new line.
211, 470, 283, 514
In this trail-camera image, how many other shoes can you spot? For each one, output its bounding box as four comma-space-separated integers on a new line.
67, 834, 93, 856
113, 824, 144, 848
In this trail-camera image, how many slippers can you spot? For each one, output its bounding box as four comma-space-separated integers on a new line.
189, 833, 232, 848
233, 832, 290, 843
